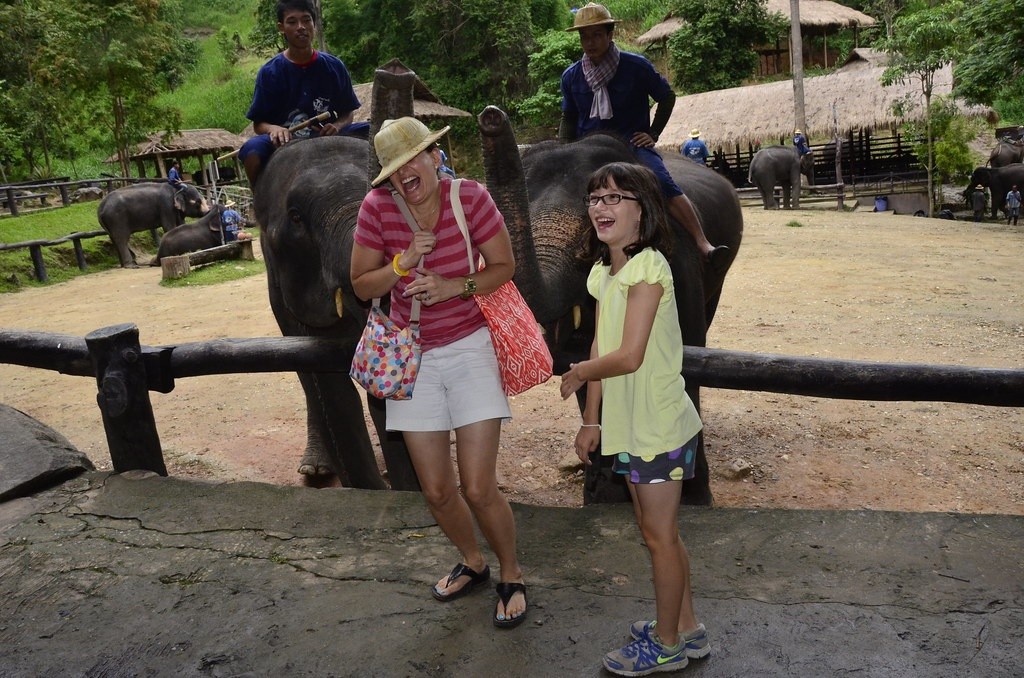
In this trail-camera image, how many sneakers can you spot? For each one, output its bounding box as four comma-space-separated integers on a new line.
603, 624, 689, 677
630, 620, 711, 659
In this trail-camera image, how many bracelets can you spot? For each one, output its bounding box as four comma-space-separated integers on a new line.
581, 424, 601, 430
393, 253, 409, 276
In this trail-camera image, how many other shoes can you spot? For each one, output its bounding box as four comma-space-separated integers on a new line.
709, 245, 729, 271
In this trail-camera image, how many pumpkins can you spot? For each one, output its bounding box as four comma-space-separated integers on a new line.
238, 233, 253, 239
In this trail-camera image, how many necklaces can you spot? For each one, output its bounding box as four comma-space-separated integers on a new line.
422, 201, 439, 221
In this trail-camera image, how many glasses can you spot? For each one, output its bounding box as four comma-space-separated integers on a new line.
583, 193, 637, 206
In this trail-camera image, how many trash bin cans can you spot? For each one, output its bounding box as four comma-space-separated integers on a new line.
726, 167, 745, 188
875, 196, 888, 211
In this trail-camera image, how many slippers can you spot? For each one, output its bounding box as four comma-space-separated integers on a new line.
494, 583, 527, 626
432, 563, 490, 601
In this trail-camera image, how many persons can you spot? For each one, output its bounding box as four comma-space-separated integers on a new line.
238, 0, 370, 191
681, 129, 709, 165
754, 141, 761, 150
168, 160, 187, 196
972, 184, 986, 222
559, 2, 731, 280
1006, 185, 1021, 226
439, 150, 455, 179
793, 129, 810, 155
560, 162, 712, 677
221, 200, 240, 243
350, 116, 527, 628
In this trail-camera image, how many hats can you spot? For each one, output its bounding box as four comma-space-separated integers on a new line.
689, 129, 701, 137
225, 200, 235, 207
975, 184, 984, 189
371, 115, 451, 186
795, 129, 802, 134
565, 2, 623, 31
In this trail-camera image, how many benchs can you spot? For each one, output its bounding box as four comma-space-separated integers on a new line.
161, 236, 258, 280
0, 193, 52, 207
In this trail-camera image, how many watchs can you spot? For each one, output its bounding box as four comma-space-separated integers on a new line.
461, 276, 476, 299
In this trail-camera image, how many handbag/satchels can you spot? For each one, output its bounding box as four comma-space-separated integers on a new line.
472, 279, 553, 396
349, 306, 422, 399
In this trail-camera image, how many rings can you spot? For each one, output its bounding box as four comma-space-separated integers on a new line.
424, 292, 430, 300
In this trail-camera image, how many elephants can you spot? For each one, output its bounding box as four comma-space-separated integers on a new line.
476, 105, 744, 508
748, 144, 815, 210
97, 183, 256, 269
955, 141, 1024, 220
255, 57, 426, 492
